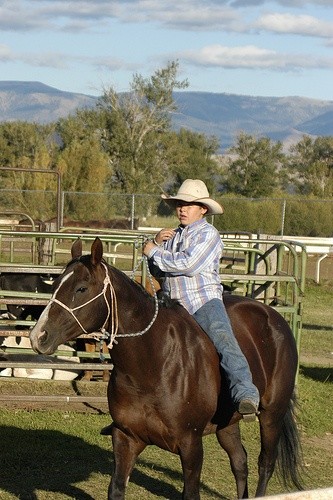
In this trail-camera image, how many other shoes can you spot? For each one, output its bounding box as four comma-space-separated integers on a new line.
239, 399, 260, 423
99, 423, 112, 436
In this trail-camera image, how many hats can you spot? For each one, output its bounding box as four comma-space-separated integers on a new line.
160, 178, 224, 215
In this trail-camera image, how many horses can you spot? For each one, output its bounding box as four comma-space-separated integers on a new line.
29, 236, 314, 496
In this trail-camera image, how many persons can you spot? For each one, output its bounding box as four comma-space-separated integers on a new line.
143, 179, 260, 422
